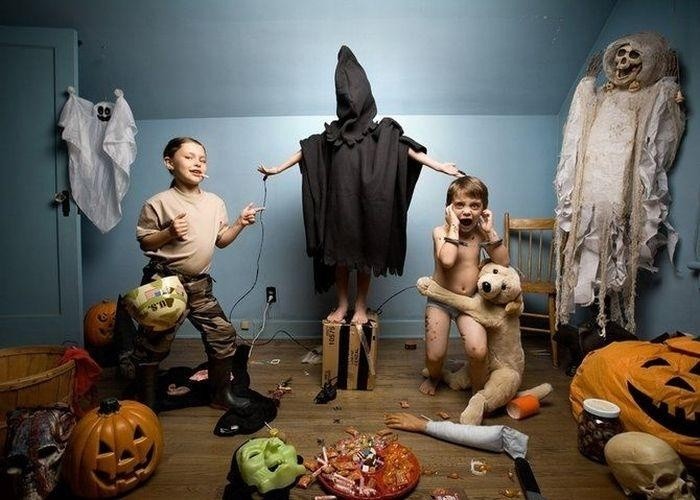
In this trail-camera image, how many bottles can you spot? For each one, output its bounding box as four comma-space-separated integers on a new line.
577, 398, 626, 465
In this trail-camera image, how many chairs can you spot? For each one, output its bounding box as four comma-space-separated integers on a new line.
504, 212, 568, 367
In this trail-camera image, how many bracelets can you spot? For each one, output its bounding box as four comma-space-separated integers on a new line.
490, 239, 503, 247
444, 237, 459, 244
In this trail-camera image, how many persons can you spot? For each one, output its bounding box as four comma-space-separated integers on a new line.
419, 175, 511, 396
131, 137, 266, 415
256, 149, 459, 324
385, 411, 529, 460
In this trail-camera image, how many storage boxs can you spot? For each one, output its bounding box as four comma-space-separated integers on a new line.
321, 309, 379, 391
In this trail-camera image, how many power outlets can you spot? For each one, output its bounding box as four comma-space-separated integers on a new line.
266, 287, 276, 304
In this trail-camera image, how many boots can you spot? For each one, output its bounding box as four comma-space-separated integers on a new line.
207, 356, 250, 411
132, 360, 158, 409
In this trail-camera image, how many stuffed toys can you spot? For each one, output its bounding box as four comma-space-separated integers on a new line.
416, 258, 553, 425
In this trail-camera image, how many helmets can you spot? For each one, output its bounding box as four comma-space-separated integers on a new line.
120, 276, 189, 329
